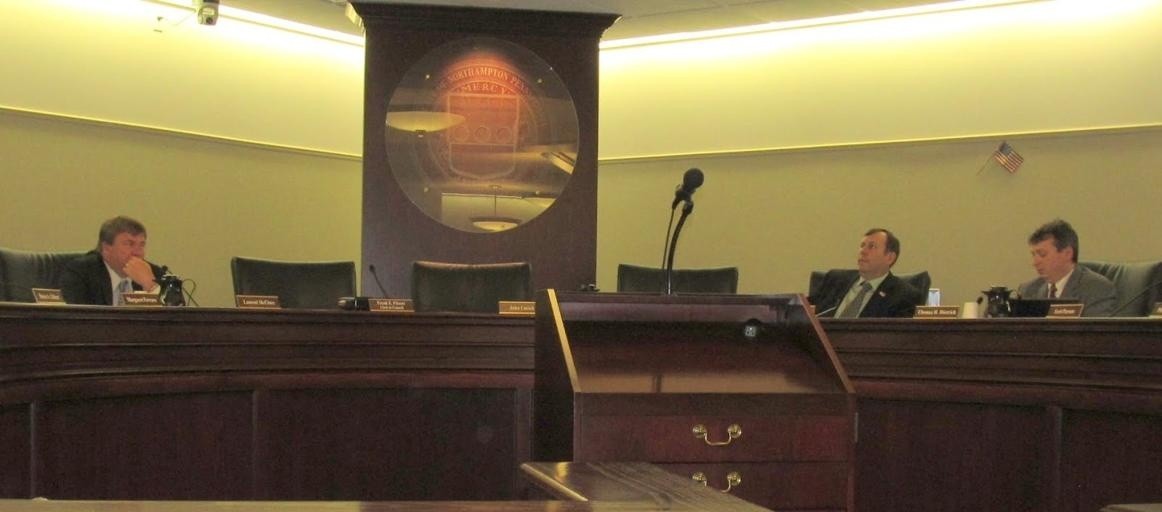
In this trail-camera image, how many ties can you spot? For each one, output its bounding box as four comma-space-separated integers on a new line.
1050, 283, 1058, 300
118, 280, 128, 306
837, 283, 872, 318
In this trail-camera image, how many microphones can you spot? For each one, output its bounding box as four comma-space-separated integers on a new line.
818, 292, 847, 319
672, 166, 703, 211
1076, 292, 1115, 316
367, 262, 393, 300
160, 260, 197, 309
1111, 280, 1162, 317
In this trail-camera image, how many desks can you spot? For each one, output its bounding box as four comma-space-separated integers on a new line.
3, 305, 1162, 512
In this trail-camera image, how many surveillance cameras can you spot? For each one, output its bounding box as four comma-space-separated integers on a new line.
193, 0, 221, 27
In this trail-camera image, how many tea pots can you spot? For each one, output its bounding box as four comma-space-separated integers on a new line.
982, 286, 1021, 318
152, 271, 196, 306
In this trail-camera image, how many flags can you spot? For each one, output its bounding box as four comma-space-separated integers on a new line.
992, 141, 1024, 175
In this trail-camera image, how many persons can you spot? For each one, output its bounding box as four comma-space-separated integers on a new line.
1014, 216, 1118, 317
808, 229, 920, 317
60, 216, 168, 306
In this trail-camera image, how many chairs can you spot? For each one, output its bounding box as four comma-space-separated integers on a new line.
1084, 261, 1162, 316
808, 270, 931, 308
618, 263, 740, 294
3, 248, 85, 302
412, 261, 532, 313
230, 256, 356, 308
